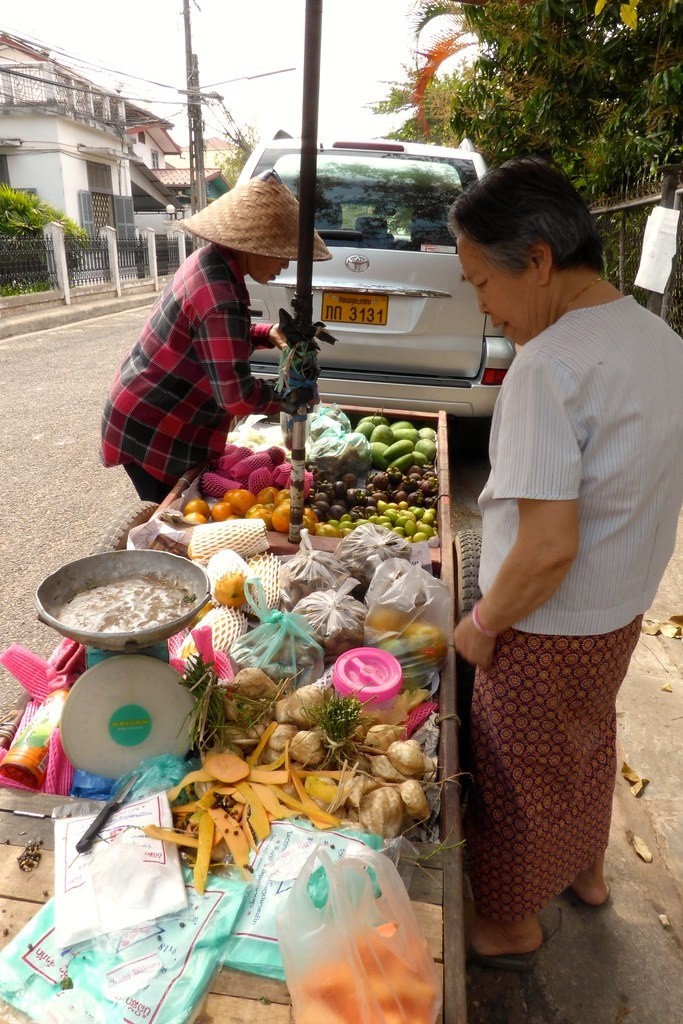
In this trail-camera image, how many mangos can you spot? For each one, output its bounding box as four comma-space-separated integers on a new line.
177, 518, 281, 661
354, 416, 437, 471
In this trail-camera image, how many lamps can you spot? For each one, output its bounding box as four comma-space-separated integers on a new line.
165, 204, 176, 216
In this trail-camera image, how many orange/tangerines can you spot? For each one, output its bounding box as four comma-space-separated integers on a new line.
183, 486, 340, 539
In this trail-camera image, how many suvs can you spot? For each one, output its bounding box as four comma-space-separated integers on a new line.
233, 132, 518, 460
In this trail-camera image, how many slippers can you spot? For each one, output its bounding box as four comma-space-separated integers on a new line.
463, 939, 539, 972
564, 883, 610, 908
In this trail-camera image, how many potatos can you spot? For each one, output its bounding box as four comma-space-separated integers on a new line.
222, 666, 435, 840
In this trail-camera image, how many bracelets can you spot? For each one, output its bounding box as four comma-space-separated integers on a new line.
472, 601, 503, 639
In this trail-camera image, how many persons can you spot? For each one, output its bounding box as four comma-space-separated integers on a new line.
445, 158, 683, 978
101, 167, 336, 507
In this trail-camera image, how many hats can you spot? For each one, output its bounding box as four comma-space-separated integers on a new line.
180, 169, 332, 263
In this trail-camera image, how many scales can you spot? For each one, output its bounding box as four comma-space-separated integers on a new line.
33, 548, 212, 777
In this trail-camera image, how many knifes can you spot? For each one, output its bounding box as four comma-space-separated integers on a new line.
75, 771, 142, 853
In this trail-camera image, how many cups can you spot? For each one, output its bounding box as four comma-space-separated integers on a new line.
331, 648, 401, 729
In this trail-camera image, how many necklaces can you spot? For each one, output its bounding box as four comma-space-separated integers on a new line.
557, 277, 605, 319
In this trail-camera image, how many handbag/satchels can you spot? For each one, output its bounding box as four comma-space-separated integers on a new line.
229, 522, 452, 693
274, 843, 441, 1023
225, 399, 374, 483
